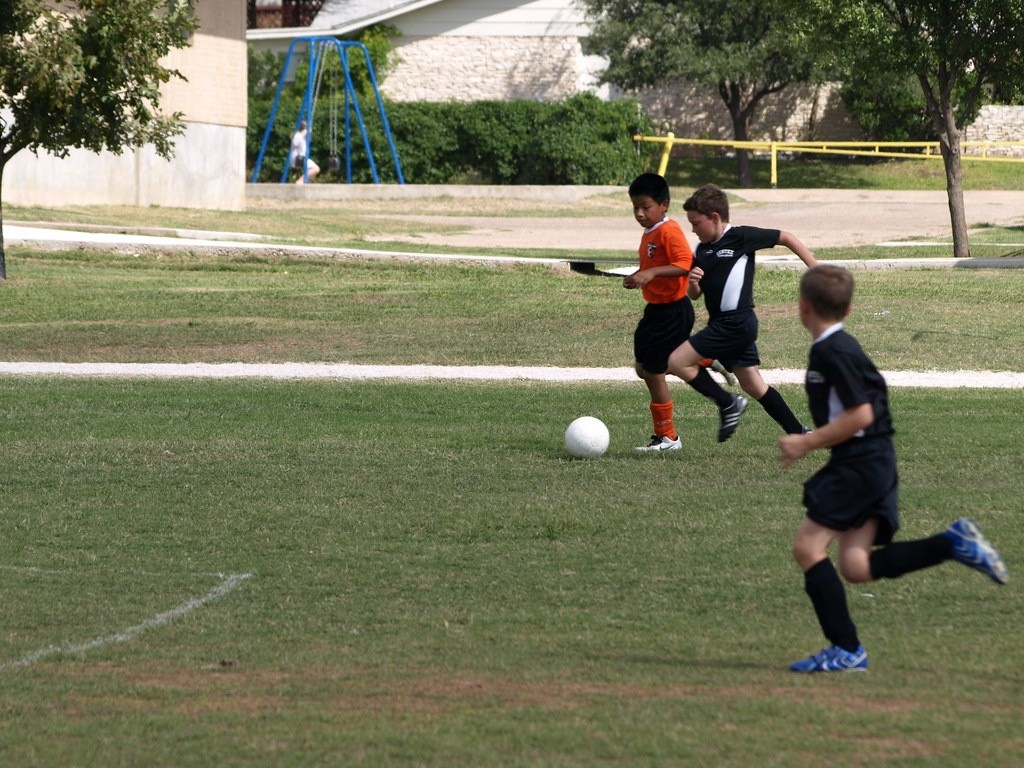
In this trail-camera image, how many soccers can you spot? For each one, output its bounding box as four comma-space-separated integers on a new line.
564, 416, 610, 458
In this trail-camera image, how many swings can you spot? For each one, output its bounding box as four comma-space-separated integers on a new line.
295, 46, 329, 170
327, 51, 339, 172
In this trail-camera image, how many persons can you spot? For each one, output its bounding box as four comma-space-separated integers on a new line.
667, 183, 820, 441
623, 173, 734, 453
289, 120, 321, 185
777, 265, 1008, 675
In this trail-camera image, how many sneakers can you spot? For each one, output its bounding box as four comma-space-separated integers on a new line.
718, 393, 748, 442
789, 644, 868, 673
635, 435, 682, 451
942, 516, 1009, 585
802, 424, 813, 434
